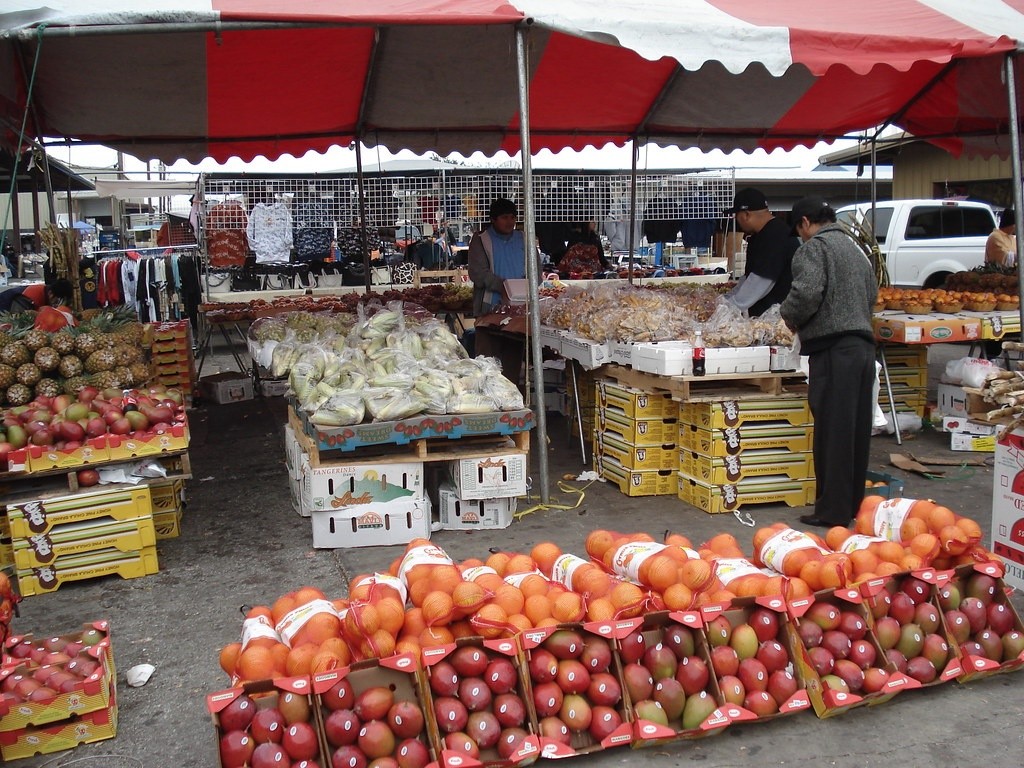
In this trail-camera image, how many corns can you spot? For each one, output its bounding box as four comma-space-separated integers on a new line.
273, 310, 524, 425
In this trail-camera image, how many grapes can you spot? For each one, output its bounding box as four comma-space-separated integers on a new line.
416, 282, 475, 304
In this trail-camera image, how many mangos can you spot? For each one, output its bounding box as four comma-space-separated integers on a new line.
215, 606, 797, 768
0, 628, 105, 702
0, 383, 182, 488
783, 572, 1024, 699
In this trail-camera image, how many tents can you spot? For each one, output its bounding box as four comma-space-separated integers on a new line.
0, 1, 1023, 511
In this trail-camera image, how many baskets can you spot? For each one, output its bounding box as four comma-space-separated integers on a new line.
885, 299, 906, 309
900, 304, 932, 315
197, 284, 474, 322
935, 303, 964, 314
996, 301, 1020, 311
969, 303, 998, 312
870, 302, 887, 312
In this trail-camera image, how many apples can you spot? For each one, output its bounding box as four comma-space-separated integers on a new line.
200, 296, 349, 315
875, 287, 1019, 306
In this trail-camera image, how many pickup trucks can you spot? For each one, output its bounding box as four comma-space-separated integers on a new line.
833, 199, 1017, 291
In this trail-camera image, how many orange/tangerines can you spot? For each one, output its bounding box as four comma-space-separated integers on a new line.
219, 495, 1001, 688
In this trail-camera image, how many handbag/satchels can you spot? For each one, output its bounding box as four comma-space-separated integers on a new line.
231, 278, 260, 292
318, 268, 342, 287
343, 265, 365, 286
256, 274, 290, 290
292, 269, 317, 289
200, 273, 232, 293
371, 265, 392, 285
392, 261, 418, 284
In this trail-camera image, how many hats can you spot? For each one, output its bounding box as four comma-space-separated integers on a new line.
787, 198, 829, 236
999, 207, 1016, 227
723, 188, 767, 215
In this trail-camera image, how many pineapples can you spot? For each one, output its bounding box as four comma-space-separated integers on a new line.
0, 305, 149, 405
944, 261, 1019, 295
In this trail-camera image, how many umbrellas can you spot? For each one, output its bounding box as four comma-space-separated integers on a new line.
73, 221, 95, 241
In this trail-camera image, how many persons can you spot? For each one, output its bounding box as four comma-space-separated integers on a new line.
467, 198, 542, 389
0, 278, 74, 312
722, 186, 801, 318
43, 251, 57, 287
604, 198, 642, 263
563, 217, 615, 271
977, 207, 1019, 360
779, 198, 879, 527
432, 218, 458, 258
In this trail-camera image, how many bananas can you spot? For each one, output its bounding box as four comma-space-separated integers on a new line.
541, 291, 794, 348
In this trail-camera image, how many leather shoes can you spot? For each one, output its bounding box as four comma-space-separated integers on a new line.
800, 514, 833, 528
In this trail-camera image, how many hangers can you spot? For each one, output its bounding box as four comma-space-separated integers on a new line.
102, 244, 193, 262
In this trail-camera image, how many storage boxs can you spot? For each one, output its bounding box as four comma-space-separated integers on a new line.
280, 425, 527, 550
0, 619, 118, 762
205, 559, 1024, 768
867, 305, 1024, 596
198, 370, 254, 405
526, 317, 822, 516
258, 371, 289, 398
151, 318, 197, 409
0, 452, 183, 596
